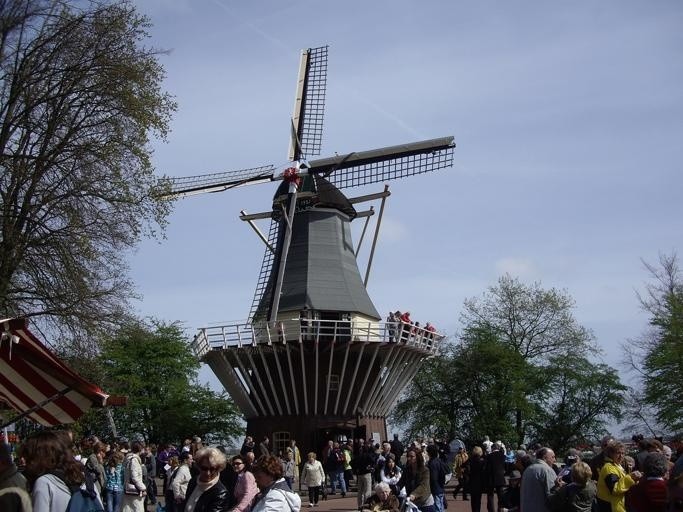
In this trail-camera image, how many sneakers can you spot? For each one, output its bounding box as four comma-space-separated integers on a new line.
308, 503, 319, 507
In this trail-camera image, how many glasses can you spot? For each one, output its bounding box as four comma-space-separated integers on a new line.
231, 462, 244, 466
200, 465, 219, 472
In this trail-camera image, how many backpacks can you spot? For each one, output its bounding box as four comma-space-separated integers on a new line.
334, 448, 346, 462
66, 487, 105, 512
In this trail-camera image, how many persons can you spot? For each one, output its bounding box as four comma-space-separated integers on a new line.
299, 305, 309, 340
384, 310, 436, 351
1, 426, 302, 512
301, 452, 325, 507
324, 434, 682, 512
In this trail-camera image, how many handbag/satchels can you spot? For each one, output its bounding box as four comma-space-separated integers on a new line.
124, 483, 140, 497
322, 485, 328, 500
